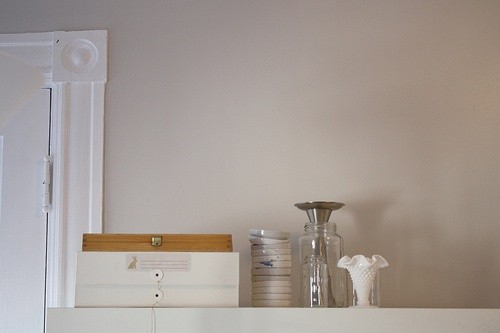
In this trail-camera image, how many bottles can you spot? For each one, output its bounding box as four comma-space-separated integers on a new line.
302, 255, 328, 308
298, 222, 348, 308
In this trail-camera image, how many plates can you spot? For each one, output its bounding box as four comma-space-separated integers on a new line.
247, 227, 292, 307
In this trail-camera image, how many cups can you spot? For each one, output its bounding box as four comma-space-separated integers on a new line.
348, 266, 383, 309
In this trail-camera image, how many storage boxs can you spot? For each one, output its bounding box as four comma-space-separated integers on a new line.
73, 233, 240, 307
